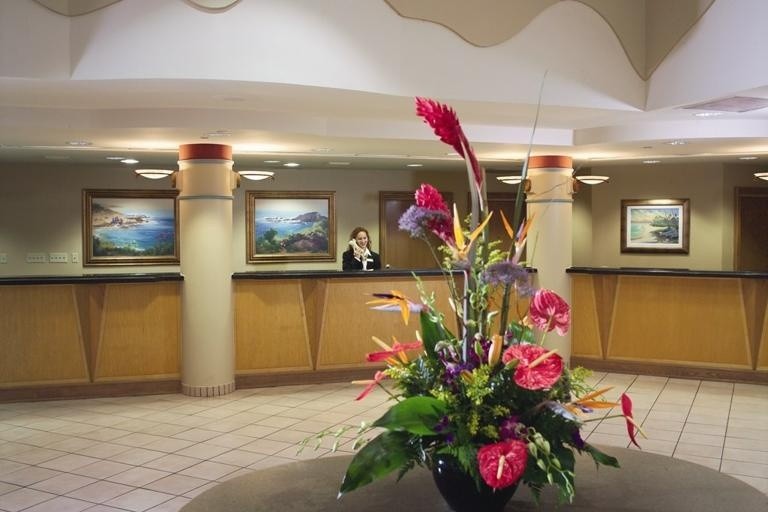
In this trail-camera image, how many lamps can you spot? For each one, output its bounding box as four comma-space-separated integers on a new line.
237, 171, 274, 188
496, 176, 531, 192
134, 170, 176, 188
573, 176, 610, 192
754, 173, 768, 181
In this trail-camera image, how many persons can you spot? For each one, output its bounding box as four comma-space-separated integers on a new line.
343, 226, 381, 271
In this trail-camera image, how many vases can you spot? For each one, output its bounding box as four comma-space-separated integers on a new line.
431, 455, 519, 512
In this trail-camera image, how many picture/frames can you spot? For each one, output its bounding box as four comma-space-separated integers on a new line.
82, 188, 180, 267
245, 190, 336, 264
621, 198, 690, 255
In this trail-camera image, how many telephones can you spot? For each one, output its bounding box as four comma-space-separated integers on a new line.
351, 239, 364, 254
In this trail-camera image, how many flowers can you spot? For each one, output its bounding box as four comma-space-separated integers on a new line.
295, 52, 646, 505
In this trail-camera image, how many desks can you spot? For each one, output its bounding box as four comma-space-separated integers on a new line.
178, 444, 768, 512
